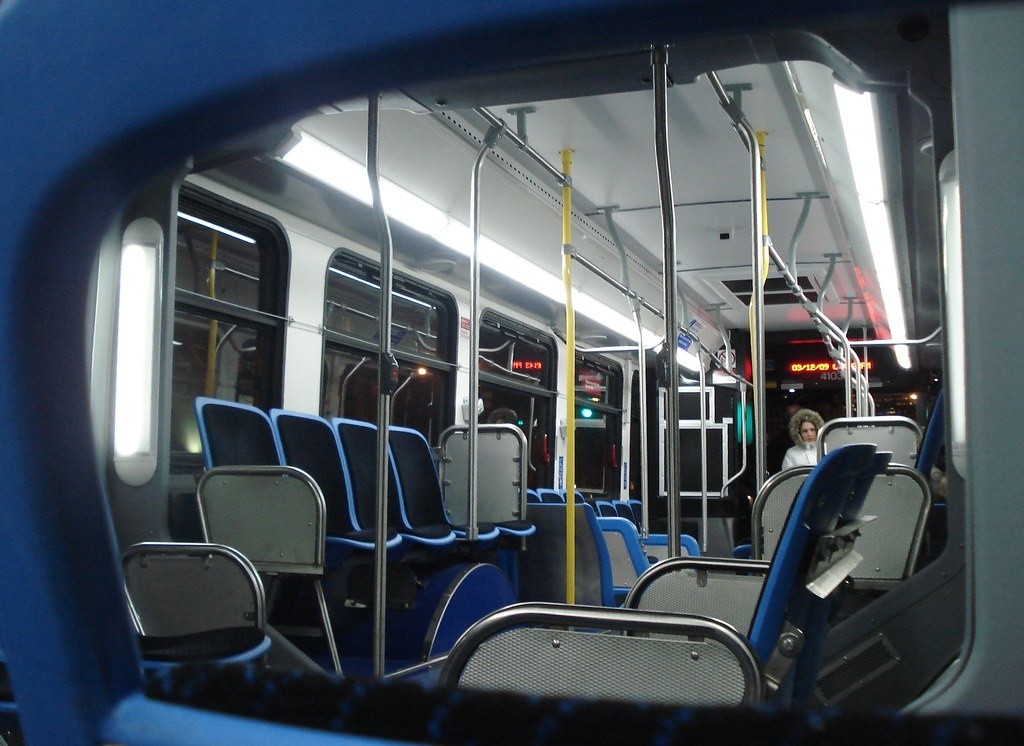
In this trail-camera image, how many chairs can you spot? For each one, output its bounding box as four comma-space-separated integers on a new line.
122, 385, 952, 713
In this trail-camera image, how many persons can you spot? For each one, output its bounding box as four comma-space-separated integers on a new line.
487, 408, 517, 424
782, 409, 825, 471
